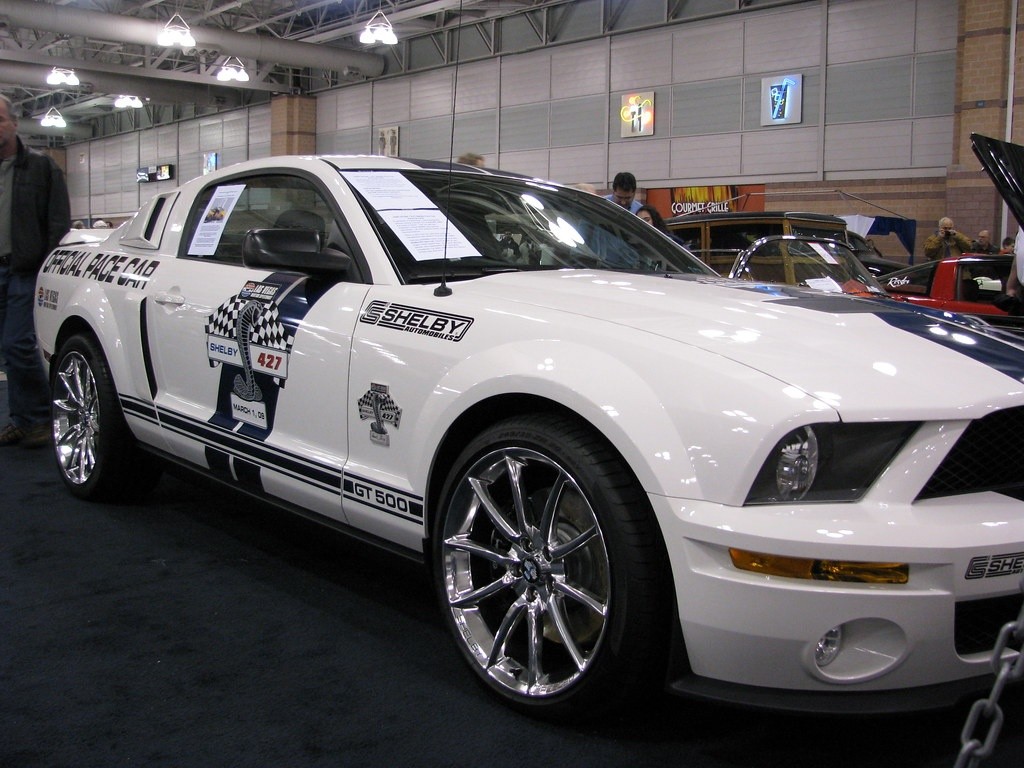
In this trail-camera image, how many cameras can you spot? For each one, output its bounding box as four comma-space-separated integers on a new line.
944, 231, 952, 237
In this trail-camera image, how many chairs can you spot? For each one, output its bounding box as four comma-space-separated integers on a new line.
243, 228, 359, 284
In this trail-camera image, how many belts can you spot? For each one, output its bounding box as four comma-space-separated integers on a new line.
0, 255, 10, 266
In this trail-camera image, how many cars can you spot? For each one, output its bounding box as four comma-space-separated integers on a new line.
869, 253, 1024, 318
848, 228, 912, 282
659, 209, 848, 286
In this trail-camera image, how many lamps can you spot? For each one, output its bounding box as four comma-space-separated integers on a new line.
158, 14, 195, 48
360, 9, 398, 45
218, 56, 249, 82
38, 93, 66, 128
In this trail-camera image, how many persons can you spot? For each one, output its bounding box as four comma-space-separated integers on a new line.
455, 153, 501, 259
501, 230, 542, 267
602, 172, 643, 216
1006, 224, 1023, 316
635, 205, 691, 253
924, 217, 973, 261
974, 229, 999, 254
71, 219, 114, 229
1, 94, 71, 450
999, 237, 1016, 255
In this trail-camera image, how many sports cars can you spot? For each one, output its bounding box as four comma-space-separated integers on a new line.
31, 157, 1023, 731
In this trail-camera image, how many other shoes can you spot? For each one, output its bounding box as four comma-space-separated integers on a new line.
0, 422, 23, 447
24, 425, 50, 447
